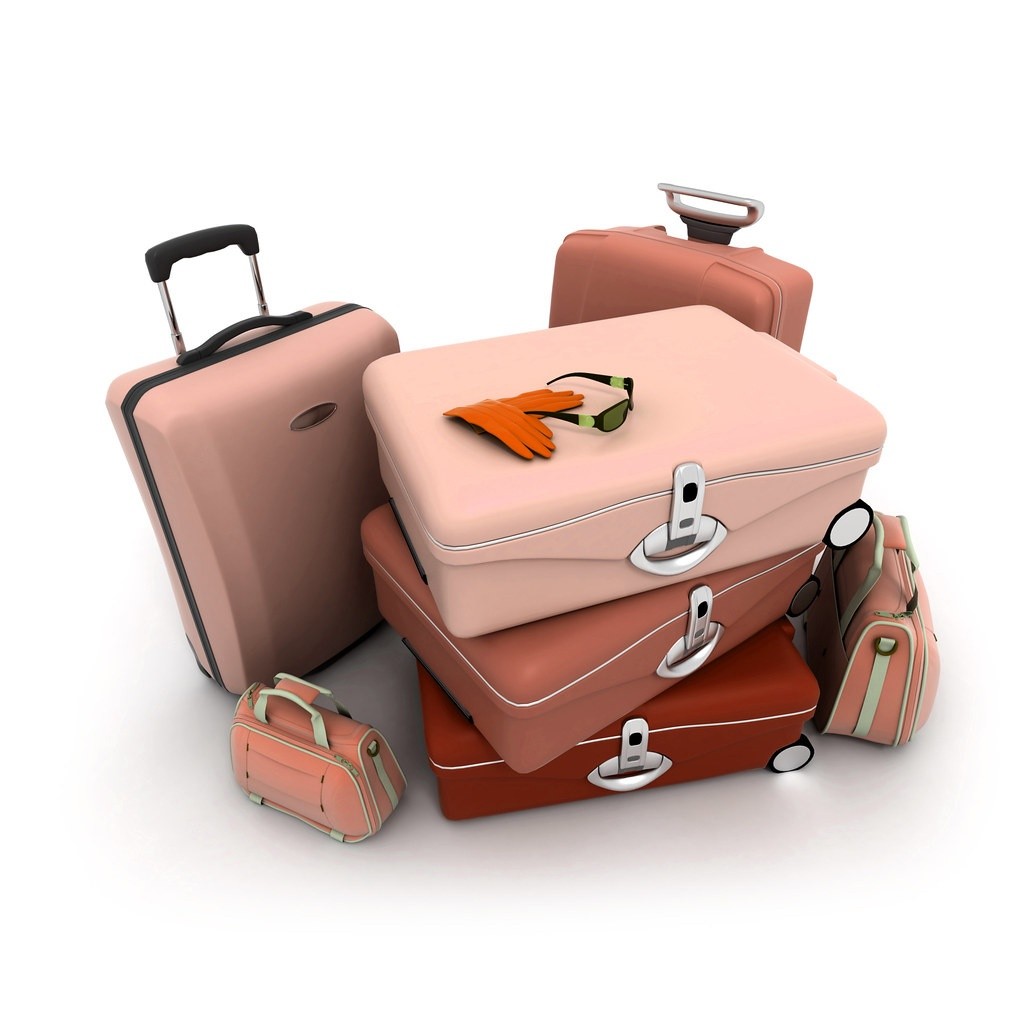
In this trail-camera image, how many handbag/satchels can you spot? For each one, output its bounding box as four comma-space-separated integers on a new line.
803, 510, 941, 747
229, 672, 408, 843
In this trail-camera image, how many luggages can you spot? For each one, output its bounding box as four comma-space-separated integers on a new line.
105, 225, 400, 698
549, 182, 814, 352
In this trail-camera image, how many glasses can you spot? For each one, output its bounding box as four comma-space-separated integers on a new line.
524, 372, 635, 432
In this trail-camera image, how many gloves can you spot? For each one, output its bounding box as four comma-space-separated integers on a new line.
442, 388, 584, 460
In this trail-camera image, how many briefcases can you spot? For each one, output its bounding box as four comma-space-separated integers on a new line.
363, 305, 887, 821
